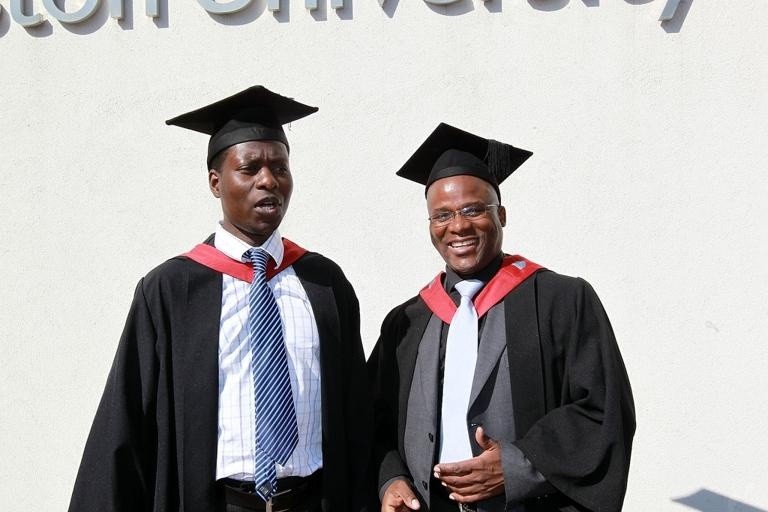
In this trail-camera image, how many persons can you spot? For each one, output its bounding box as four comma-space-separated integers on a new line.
370, 120, 634, 511
72, 85, 364, 510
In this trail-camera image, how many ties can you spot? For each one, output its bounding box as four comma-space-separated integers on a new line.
242, 248, 299, 501
438, 278, 484, 464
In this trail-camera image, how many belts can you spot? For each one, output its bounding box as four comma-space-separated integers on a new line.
224, 482, 300, 512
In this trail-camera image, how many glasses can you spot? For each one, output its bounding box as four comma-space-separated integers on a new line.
428, 204, 496, 221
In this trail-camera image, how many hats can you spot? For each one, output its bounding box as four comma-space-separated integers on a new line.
396, 122, 533, 203
165, 85, 318, 164
